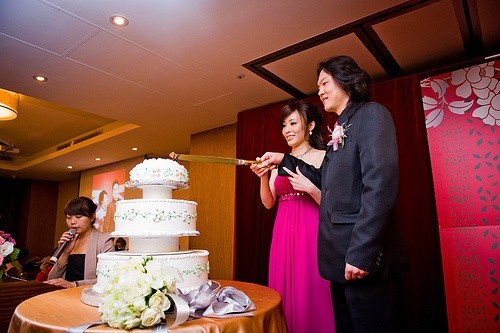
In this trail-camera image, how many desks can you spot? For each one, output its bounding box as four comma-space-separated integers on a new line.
8, 280, 285, 332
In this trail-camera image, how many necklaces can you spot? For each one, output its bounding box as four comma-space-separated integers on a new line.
78, 238, 89, 246
289, 146, 312, 160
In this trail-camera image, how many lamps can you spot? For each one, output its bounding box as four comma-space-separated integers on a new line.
0, 88, 20, 122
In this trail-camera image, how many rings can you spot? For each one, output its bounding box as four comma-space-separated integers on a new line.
358, 276, 363, 278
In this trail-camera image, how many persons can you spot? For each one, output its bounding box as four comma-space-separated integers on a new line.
93, 181, 124, 233
43, 196, 115, 289
35, 255, 53, 283
250, 100, 335, 333
255, 55, 411, 333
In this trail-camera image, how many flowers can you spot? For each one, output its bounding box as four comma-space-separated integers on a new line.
327, 121, 353, 152
0, 231, 20, 277
65, 257, 257, 333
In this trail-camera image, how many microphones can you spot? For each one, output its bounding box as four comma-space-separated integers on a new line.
50, 229, 76, 264
